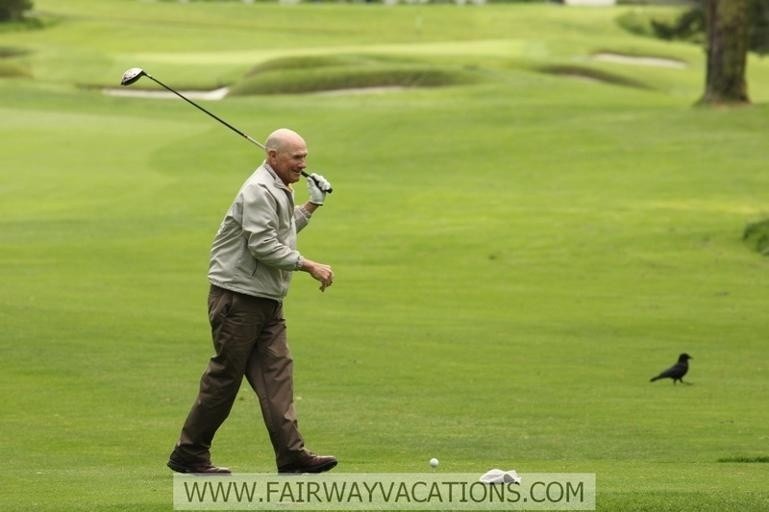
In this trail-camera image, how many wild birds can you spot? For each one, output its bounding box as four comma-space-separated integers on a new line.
649, 353, 693, 386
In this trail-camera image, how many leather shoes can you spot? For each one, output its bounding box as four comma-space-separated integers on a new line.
278, 454, 338, 478
165, 442, 233, 477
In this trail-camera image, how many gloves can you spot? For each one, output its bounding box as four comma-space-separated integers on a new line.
307, 173, 331, 208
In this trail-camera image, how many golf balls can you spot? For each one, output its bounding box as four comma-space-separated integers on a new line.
429, 457, 439, 468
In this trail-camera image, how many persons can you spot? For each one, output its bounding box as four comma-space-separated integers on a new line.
166, 127, 339, 476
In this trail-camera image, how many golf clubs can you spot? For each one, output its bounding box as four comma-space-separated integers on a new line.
120, 67, 333, 194
